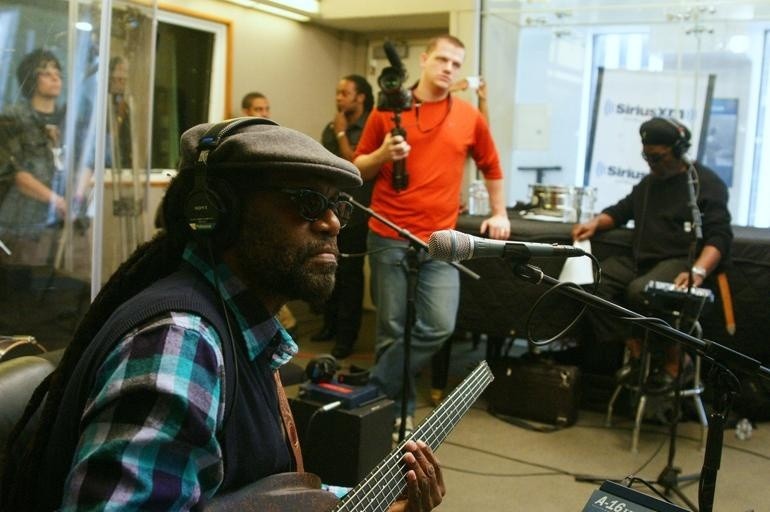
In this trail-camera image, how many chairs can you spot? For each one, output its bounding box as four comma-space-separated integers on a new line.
0, 347, 65, 457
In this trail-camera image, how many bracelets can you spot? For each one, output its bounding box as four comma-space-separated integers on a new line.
690, 264, 710, 282
334, 130, 349, 139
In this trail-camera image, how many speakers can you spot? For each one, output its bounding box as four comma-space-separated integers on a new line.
283, 380, 395, 488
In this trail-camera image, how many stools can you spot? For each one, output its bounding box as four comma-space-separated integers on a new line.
606, 312, 710, 455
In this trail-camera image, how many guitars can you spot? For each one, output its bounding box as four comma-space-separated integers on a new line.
190, 359, 495, 512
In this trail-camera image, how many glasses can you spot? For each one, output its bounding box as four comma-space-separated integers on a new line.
273, 187, 353, 229
642, 147, 672, 161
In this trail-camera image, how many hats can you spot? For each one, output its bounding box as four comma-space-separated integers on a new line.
178, 123, 363, 189
640, 120, 690, 144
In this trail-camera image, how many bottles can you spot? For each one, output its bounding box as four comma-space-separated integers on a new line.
469, 179, 488, 214
526, 186, 598, 226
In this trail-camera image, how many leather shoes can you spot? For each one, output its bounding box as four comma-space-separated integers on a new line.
311, 328, 351, 360
616, 357, 673, 393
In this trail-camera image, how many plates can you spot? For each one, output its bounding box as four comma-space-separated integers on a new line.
518, 209, 573, 224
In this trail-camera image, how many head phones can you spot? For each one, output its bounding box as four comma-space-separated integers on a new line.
164, 116, 278, 256
666, 116, 692, 159
305, 354, 371, 386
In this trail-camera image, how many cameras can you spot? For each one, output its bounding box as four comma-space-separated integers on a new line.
377, 66, 413, 111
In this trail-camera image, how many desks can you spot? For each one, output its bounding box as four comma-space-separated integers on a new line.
454, 206, 770, 416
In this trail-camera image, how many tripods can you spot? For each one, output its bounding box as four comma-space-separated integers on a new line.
574, 312, 702, 512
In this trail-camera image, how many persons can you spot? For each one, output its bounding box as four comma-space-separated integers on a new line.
32, 118, 446, 511
573, 117, 735, 393
350, 33, 515, 436
0, 47, 73, 269
312, 74, 379, 362
241, 91, 270, 119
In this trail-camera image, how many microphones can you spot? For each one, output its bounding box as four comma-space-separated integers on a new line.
429, 227, 585, 262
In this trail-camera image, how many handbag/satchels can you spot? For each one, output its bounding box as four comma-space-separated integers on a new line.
491, 352, 580, 427
